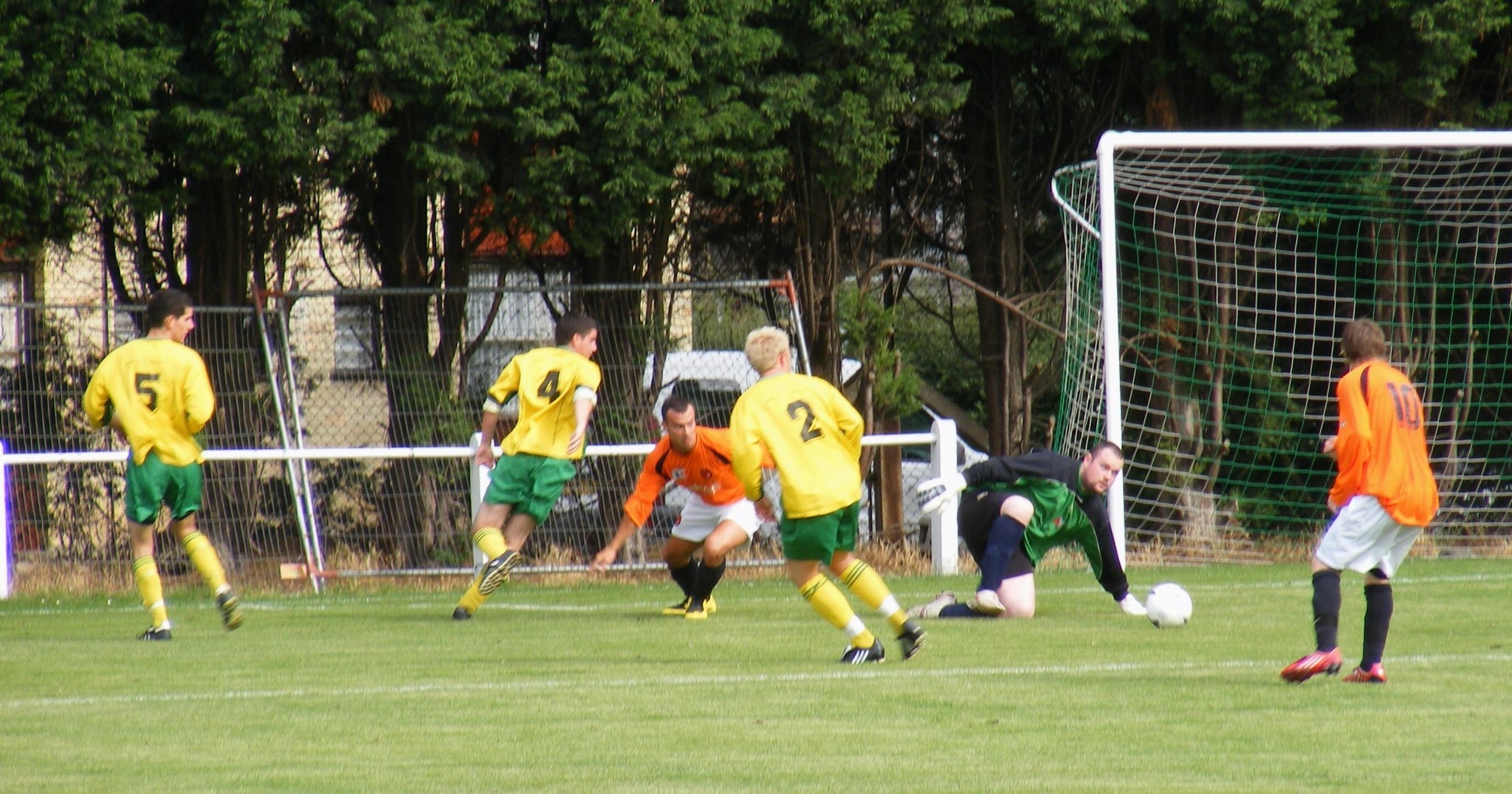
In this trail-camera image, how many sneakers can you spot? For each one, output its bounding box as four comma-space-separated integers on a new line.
972, 589, 1006, 615
895, 620, 927, 660
1282, 646, 1342, 681
138, 621, 171, 641
1343, 661, 1388, 683
662, 594, 716, 616
217, 590, 243, 630
684, 596, 707, 621
454, 606, 471, 621
839, 637, 885, 664
907, 590, 957, 621
478, 549, 522, 596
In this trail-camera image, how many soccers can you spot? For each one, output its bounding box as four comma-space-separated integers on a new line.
1145, 582, 1195, 631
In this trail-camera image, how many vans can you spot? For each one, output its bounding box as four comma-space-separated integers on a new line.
642, 349, 990, 555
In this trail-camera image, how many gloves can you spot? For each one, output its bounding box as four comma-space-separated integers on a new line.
1116, 593, 1146, 616
915, 471, 967, 513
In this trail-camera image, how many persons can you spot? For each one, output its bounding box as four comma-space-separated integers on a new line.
1279, 320, 1441, 685
450, 313, 602, 620
914, 438, 1146, 621
730, 328, 928, 666
81, 291, 246, 644
595, 398, 774, 620
